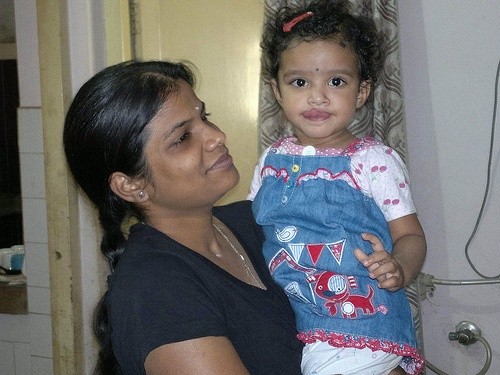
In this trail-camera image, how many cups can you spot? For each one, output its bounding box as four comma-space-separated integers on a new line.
0, 245, 25, 274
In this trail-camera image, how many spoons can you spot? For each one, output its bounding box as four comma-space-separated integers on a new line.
0, 266, 21, 274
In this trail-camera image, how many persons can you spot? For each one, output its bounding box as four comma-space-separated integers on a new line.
242, 0, 432, 375
61, 56, 307, 375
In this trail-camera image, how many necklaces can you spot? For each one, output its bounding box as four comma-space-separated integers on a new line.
211, 223, 265, 293
